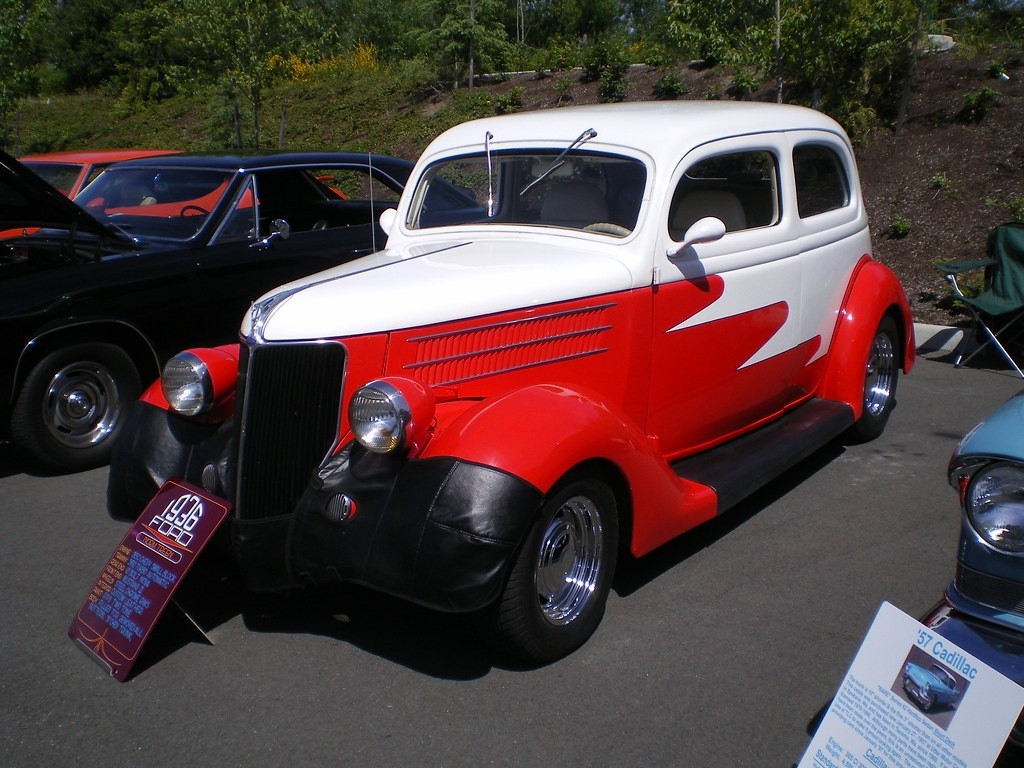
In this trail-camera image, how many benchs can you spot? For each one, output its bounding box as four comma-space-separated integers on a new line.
292, 200, 396, 227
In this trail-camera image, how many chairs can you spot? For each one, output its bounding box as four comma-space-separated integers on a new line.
541, 183, 608, 220
935, 221, 1024, 379
673, 190, 746, 229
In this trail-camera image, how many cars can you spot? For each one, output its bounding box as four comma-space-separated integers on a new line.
0, 148, 349, 239
1, 145, 490, 474
106, 100, 916, 670
916, 267, 1024, 763
904, 661, 961, 712
